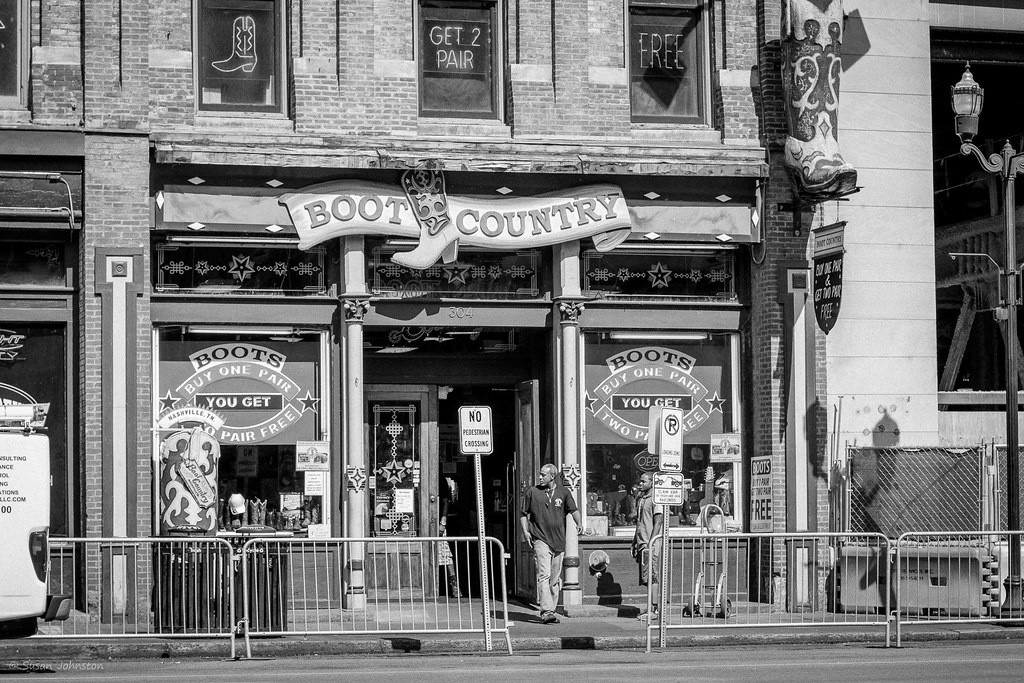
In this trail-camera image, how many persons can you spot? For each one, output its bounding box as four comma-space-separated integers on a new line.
440, 473, 462, 597
520, 463, 583, 623
631, 473, 663, 620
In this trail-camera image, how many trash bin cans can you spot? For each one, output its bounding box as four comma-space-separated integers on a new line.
224, 523, 291, 634
148, 525, 228, 634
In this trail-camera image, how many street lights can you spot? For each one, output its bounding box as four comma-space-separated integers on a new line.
951, 60, 1024, 628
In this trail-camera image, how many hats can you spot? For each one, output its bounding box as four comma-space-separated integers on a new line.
228, 493, 245, 515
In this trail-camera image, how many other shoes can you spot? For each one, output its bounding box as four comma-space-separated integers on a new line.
637, 610, 657, 621
540, 611, 560, 624
453, 588, 462, 598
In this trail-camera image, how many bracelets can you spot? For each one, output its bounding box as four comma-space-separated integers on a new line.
440, 521, 446, 525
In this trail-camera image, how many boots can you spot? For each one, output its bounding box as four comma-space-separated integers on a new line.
218, 496, 321, 533
586, 483, 730, 526
